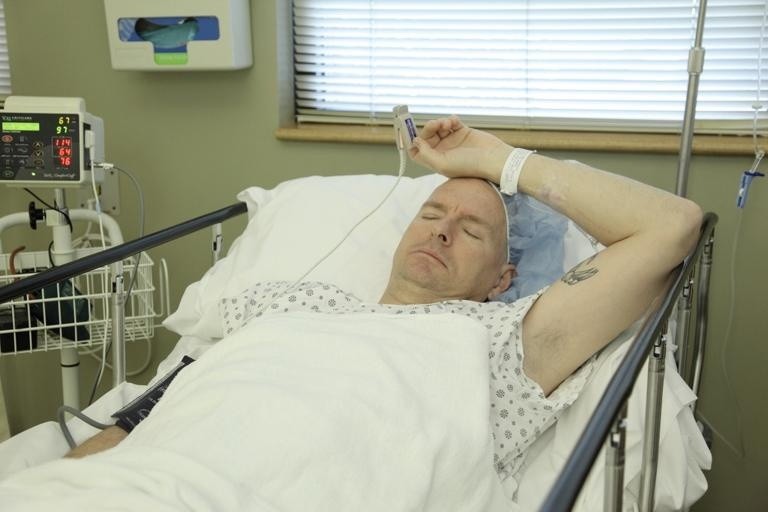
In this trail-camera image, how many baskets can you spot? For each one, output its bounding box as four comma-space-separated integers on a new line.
0, 246, 154, 356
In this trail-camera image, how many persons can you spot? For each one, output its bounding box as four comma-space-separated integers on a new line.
63, 117, 703, 483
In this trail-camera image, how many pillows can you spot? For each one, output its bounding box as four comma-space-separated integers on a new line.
164, 172, 670, 378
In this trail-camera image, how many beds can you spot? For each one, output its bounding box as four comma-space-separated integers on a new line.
0, 172, 719, 510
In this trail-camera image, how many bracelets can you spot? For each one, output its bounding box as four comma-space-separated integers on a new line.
501, 147, 536, 195
